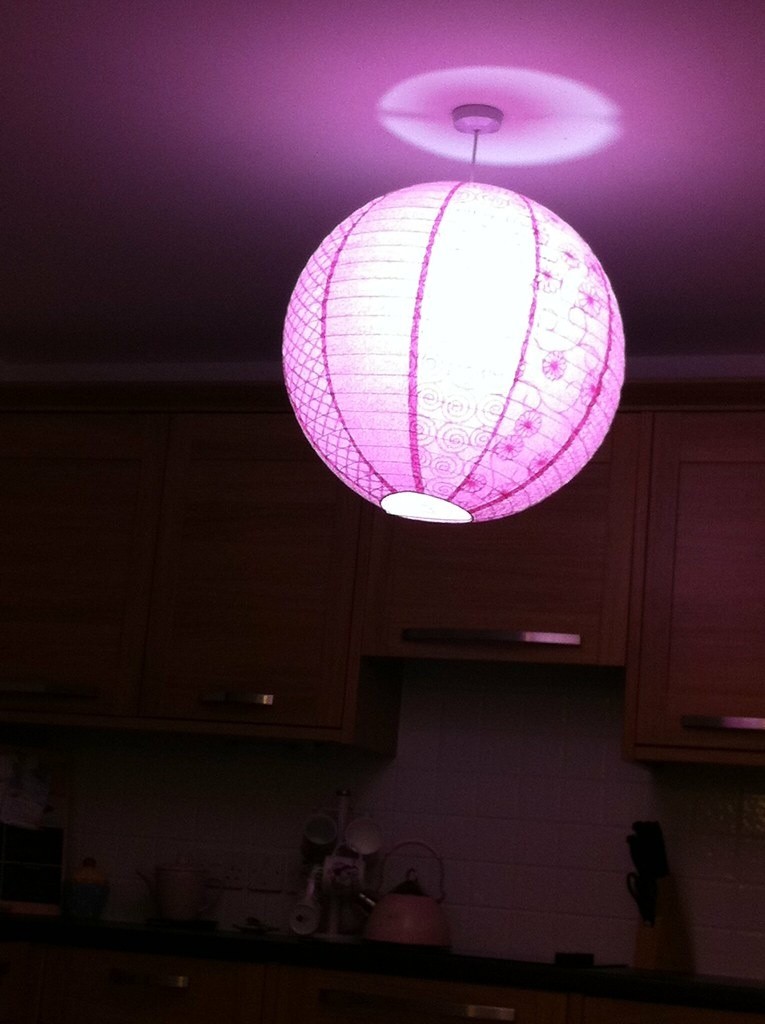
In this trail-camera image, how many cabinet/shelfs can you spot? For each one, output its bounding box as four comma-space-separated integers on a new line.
0, 410, 401, 757
623, 406, 765, 768
364, 408, 645, 665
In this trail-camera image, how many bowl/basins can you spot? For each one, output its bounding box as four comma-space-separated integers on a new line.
361, 892, 452, 946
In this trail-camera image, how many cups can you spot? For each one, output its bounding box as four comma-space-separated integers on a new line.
319, 857, 368, 900
345, 817, 383, 858
301, 807, 336, 855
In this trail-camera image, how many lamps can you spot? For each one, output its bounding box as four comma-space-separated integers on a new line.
284, 104, 627, 522
